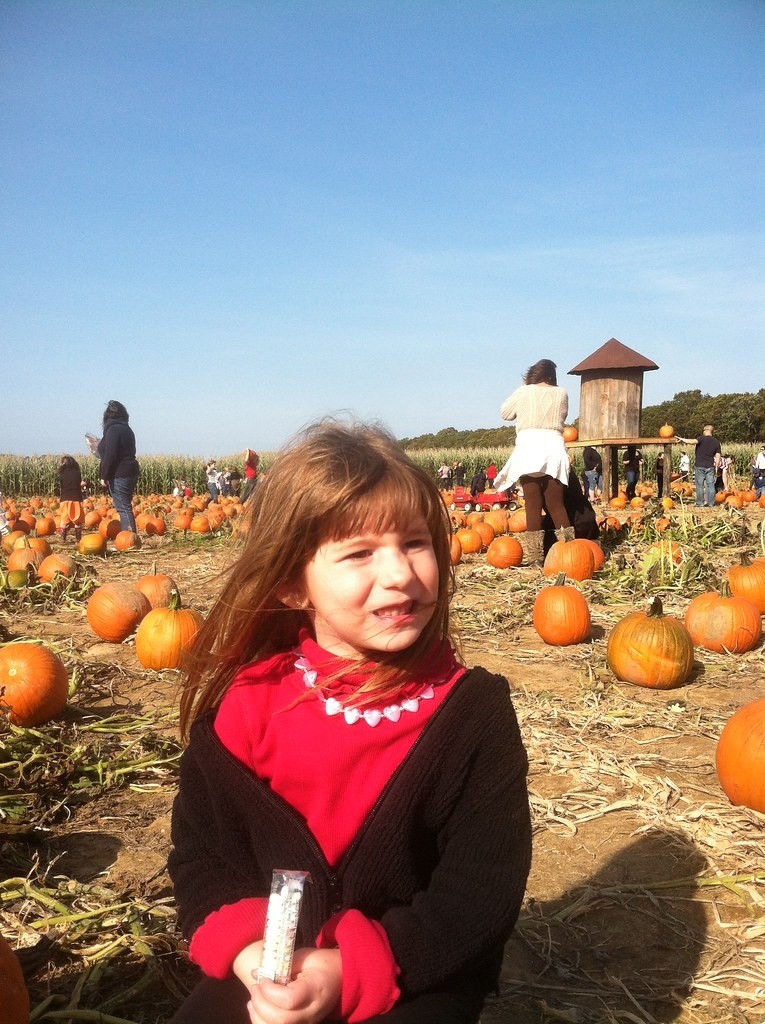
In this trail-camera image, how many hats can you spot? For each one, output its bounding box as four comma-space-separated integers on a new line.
753, 468, 761, 474
227, 473, 242, 480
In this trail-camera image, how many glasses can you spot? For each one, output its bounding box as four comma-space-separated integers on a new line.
761, 449, 765, 450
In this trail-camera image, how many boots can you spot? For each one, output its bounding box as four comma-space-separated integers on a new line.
75, 527, 82, 545
58, 528, 67, 543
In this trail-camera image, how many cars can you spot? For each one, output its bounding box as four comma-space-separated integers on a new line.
450, 486, 518, 512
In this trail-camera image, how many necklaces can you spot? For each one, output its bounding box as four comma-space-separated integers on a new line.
292, 638, 455, 728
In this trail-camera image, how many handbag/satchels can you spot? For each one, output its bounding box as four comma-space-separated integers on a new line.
437, 471, 443, 477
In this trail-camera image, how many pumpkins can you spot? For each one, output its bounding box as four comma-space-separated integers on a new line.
0, 642, 70, 726
0, 492, 250, 670
659, 422, 674, 438
562, 426, 578, 442
439, 483, 765, 816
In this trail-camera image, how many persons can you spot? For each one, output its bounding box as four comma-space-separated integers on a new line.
168, 413, 532, 1024
173, 484, 192, 499
438, 461, 468, 493
623, 447, 643, 502
674, 425, 721, 507
97, 400, 142, 548
59, 456, 87, 543
714, 452, 735, 491
748, 469, 765, 502
582, 445, 602, 503
203, 449, 258, 505
497, 359, 575, 570
469, 473, 486, 497
487, 463, 497, 488
653, 451, 665, 499
751, 446, 765, 476
678, 451, 689, 483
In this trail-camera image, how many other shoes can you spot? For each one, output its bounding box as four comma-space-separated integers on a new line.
596, 498, 602, 502
591, 498, 597, 505
